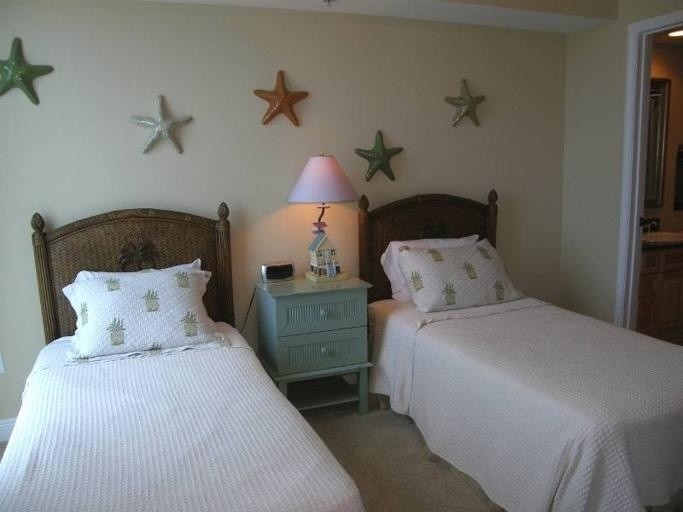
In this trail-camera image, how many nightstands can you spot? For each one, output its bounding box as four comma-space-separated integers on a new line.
253, 274, 375, 417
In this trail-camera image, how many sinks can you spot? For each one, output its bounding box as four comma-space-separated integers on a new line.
640, 232, 683, 243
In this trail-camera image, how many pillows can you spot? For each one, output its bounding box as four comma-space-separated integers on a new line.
379, 234, 522, 313
61, 258, 230, 363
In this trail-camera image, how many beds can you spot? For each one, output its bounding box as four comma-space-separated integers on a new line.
357, 188, 682, 512
0, 201, 361, 512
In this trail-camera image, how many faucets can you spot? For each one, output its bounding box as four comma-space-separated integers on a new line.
650, 216, 660, 231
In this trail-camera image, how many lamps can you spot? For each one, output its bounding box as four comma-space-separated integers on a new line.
287, 151, 362, 281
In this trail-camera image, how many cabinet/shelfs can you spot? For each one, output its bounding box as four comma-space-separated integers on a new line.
636, 249, 682, 335
